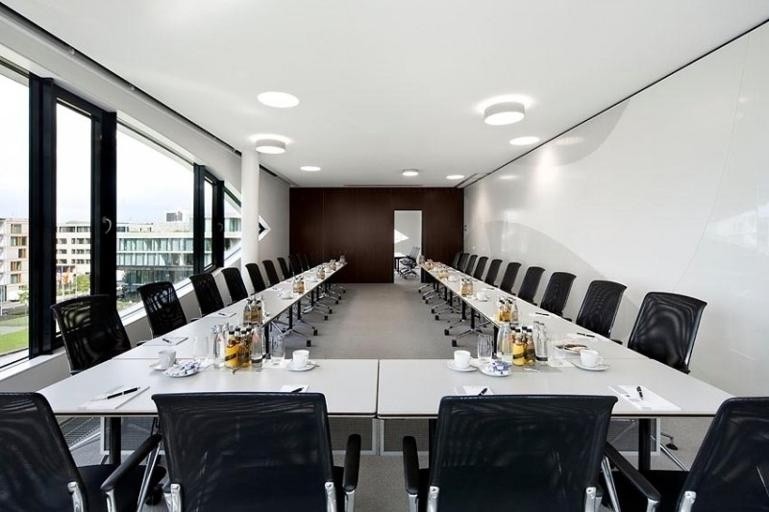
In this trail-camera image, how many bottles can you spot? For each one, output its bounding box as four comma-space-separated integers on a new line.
242, 293, 266, 326
437, 266, 449, 282
224, 330, 239, 370
238, 330, 250, 369
522, 329, 536, 366
292, 276, 305, 294
329, 260, 336, 270
316, 267, 326, 279
496, 323, 528, 366
248, 327, 263, 363
210, 322, 226, 368
425, 259, 434, 271
498, 296, 518, 321
533, 321, 549, 360
460, 278, 472, 296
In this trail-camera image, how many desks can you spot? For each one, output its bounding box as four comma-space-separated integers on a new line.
395, 255, 410, 274
417, 261, 655, 359
378, 356, 744, 476
35, 358, 378, 465
112, 261, 348, 358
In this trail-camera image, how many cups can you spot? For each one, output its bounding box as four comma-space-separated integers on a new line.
292, 350, 310, 368
454, 350, 473, 368
581, 349, 603, 367
160, 351, 176, 367
478, 334, 492, 360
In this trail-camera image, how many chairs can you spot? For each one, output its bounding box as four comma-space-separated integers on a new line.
277, 256, 335, 314
444, 261, 521, 336
628, 292, 708, 374
417, 251, 460, 292
418, 252, 470, 294
540, 271, 576, 321
190, 273, 224, 316
399, 246, 420, 279
49, 294, 149, 375
245, 263, 320, 335
450, 266, 545, 346
278, 256, 319, 336
246, 263, 288, 353
603, 395, 769, 512
221, 267, 312, 348
422, 252, 470, 303
288, 255, 339, 304
403, 394, 622, 512
0, 393, 162, 512
151, 391, 361, 512
306, 252, 346, 294
289, 255, 332, 314
307, 254, 346, 293
425, 255, 490, 303
299, 253, 342, 300
300, 254, 339, 304
444, 259, 504, 335
263, 260, 312, 348
421, 255, 478, 303
262, 260, 329, 321
435, 257, 489, 319
431, 259, 503, 320
576, 280, 627, 344
453, 262, 522, 346
431, 255, 478, 314
137, 280, 202, 339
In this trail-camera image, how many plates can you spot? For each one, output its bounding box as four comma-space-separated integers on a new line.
480, 368, 507, 377
283, 362, 314, 371
447, 360, 476, 371
574, 361, 609, 371
152, 362, 174, 370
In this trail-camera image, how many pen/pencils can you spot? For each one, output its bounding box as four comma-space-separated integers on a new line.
508, 295, 517, 302
162, 337, 171, 343
535, 312, 549, 316
637, 385, 644, 399
487, 287, 495, 291
577, 332, 595, 338
218, 312, 226, 317
477, 388, 489, 395
290, 387, 304, 393
107, 387, 138, 399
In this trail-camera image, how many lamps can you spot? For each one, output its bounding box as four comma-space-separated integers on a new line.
255, 140, 286, 154
485, 104, 525, 126
